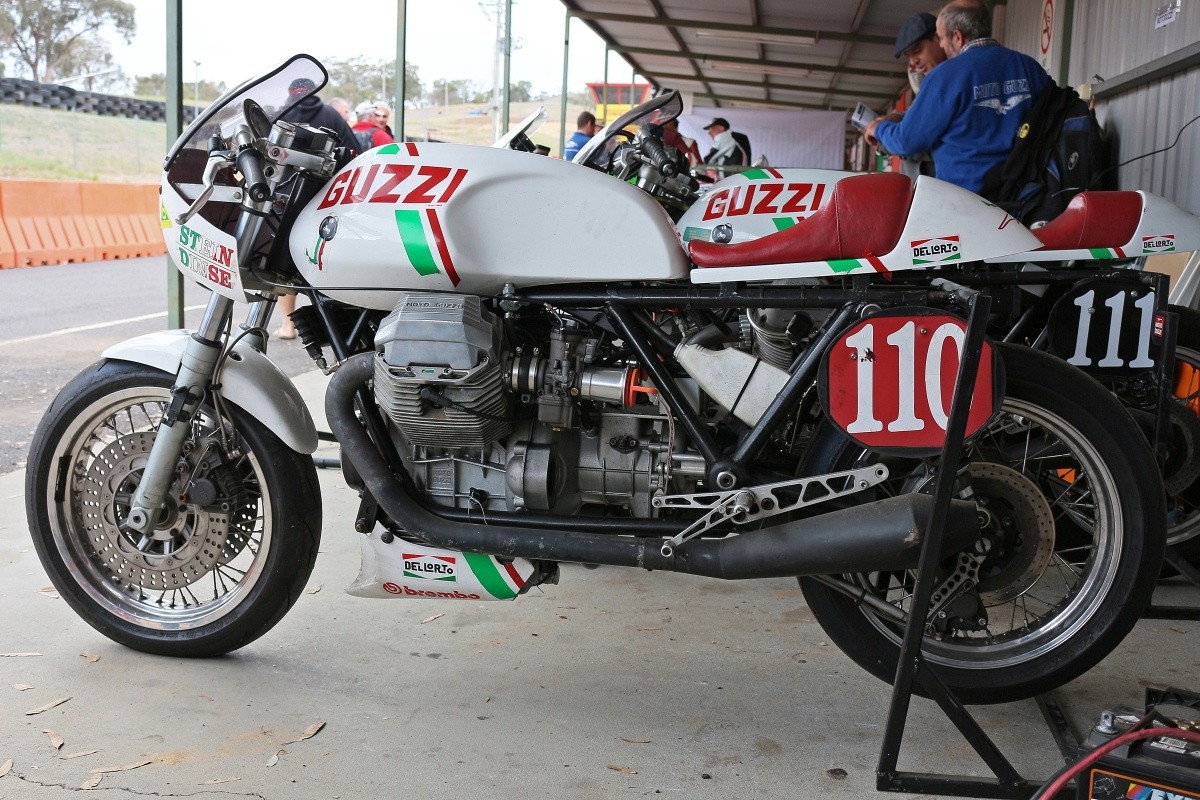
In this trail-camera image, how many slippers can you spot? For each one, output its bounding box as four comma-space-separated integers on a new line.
272, 330, 291, 339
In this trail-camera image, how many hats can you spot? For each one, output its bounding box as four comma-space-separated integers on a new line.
289, 79, 316, 96
894, 13, 936, 59
703, 118, 729, 130
356, 102, 378, 116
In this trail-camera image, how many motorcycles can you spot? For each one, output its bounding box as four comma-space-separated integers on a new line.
22, 55, 1173, 800
495, 84, 1199, 585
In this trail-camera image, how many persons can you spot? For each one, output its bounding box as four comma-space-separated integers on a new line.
562, 87, 751, 183
875, 12, 947, 180
865, 0, 1049, 193
270, 79, 394, 337
